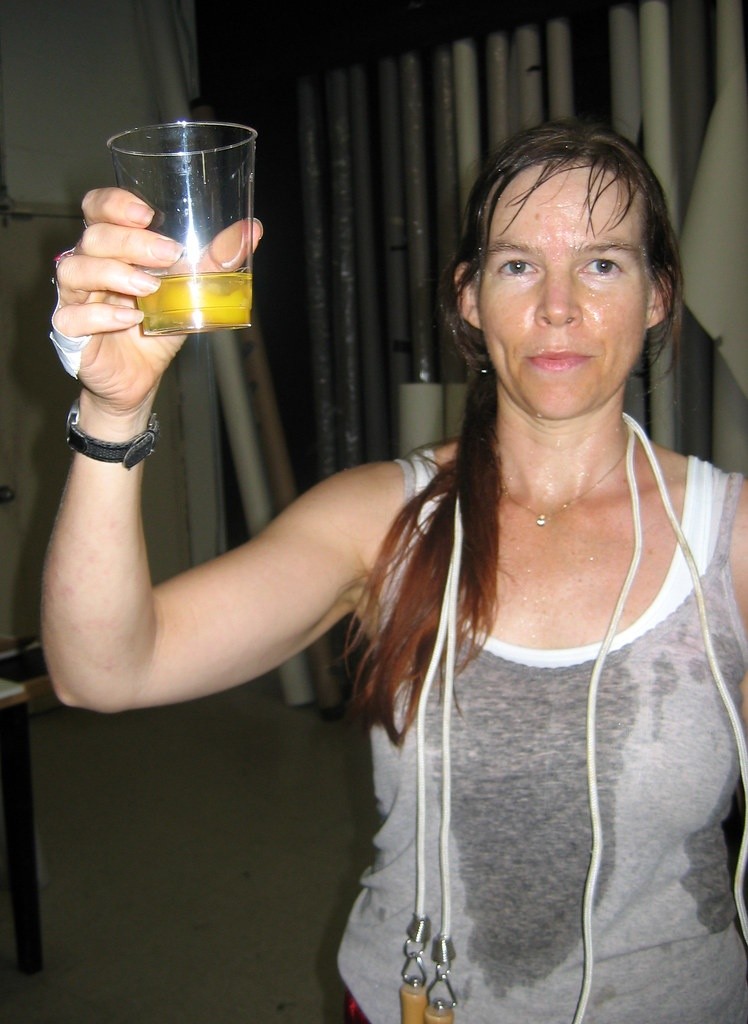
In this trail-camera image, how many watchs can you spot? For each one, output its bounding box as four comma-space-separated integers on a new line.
67, 396, 158, 470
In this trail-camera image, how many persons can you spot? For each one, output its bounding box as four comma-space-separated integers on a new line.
40, 121, 747, 1024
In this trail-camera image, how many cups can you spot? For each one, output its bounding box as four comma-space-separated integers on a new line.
105, 121, 258, 336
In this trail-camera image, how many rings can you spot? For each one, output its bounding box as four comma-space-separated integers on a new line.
54, 252, 74, 269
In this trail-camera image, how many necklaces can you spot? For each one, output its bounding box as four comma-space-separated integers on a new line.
496, 449, 627, 526
